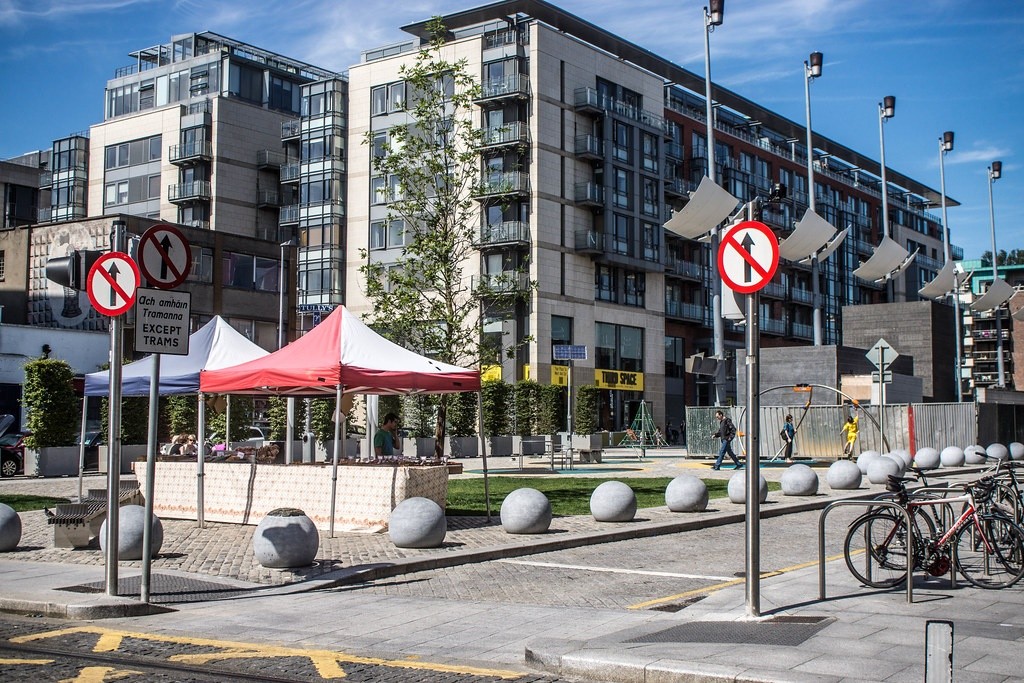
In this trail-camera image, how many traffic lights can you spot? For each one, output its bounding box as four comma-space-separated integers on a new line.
45, 249, 85, 291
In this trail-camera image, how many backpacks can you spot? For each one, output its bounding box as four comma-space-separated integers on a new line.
780, 423, 789, 440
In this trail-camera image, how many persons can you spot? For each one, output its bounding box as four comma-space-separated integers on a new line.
783, 414, 797, 463
840, 404, 860, 459
711, 411, 743, 470
167, 434, 196, 456
374, 412, 400, 456
652, 419, 687, 450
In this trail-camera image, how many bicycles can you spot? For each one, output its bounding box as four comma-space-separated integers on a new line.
843, 452, 1024, 592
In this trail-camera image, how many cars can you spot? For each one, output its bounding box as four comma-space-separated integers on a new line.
0, 414, 108, 478
160, 425, 266, 462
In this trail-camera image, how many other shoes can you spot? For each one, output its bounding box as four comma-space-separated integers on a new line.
711, 466, 720, 470
848, 453, 852, 457
734, 463, 742, 470
843, 449, 847, 454
786, 460, 793, 464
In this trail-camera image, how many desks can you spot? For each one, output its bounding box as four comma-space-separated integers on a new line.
131, 461, 462, 533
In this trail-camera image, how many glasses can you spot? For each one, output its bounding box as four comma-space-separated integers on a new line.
190, 439, 195, 442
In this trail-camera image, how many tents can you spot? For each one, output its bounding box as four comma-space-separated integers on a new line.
78, 314, 295, 526
198, 306, 492, 538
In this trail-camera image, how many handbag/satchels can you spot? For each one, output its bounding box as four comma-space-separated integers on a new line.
212, 441, 225, 452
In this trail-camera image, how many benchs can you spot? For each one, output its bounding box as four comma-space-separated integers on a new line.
48, 480, 141, 547
